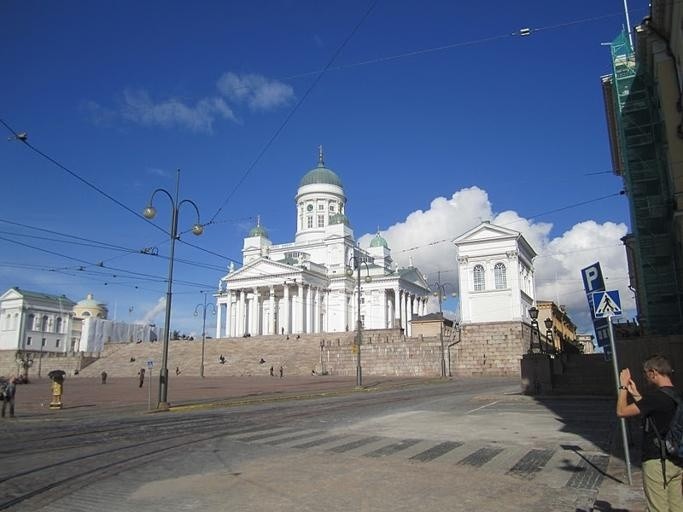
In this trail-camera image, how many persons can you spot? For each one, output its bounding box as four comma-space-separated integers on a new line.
270, 366, 274, 376
139, 372, 145, 388
280, 367, 283, 377
616, 355, 683, 512
0, 375, 17, 418
101, 371, 107, 383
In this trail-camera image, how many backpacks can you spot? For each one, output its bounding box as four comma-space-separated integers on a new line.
658, 386, 683, 462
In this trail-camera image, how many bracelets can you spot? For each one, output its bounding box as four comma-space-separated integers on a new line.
619, 386, 628, 391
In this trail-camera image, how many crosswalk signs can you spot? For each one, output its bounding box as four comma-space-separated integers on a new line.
592, 291, 621, 319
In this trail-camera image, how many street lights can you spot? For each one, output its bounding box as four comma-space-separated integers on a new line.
194, 293, 215, 377
424, 272, 457, 380
529, 306, 555, 356
347, 243, 372, 388
144, 168, 204, 409
35, 313, 52, 377
